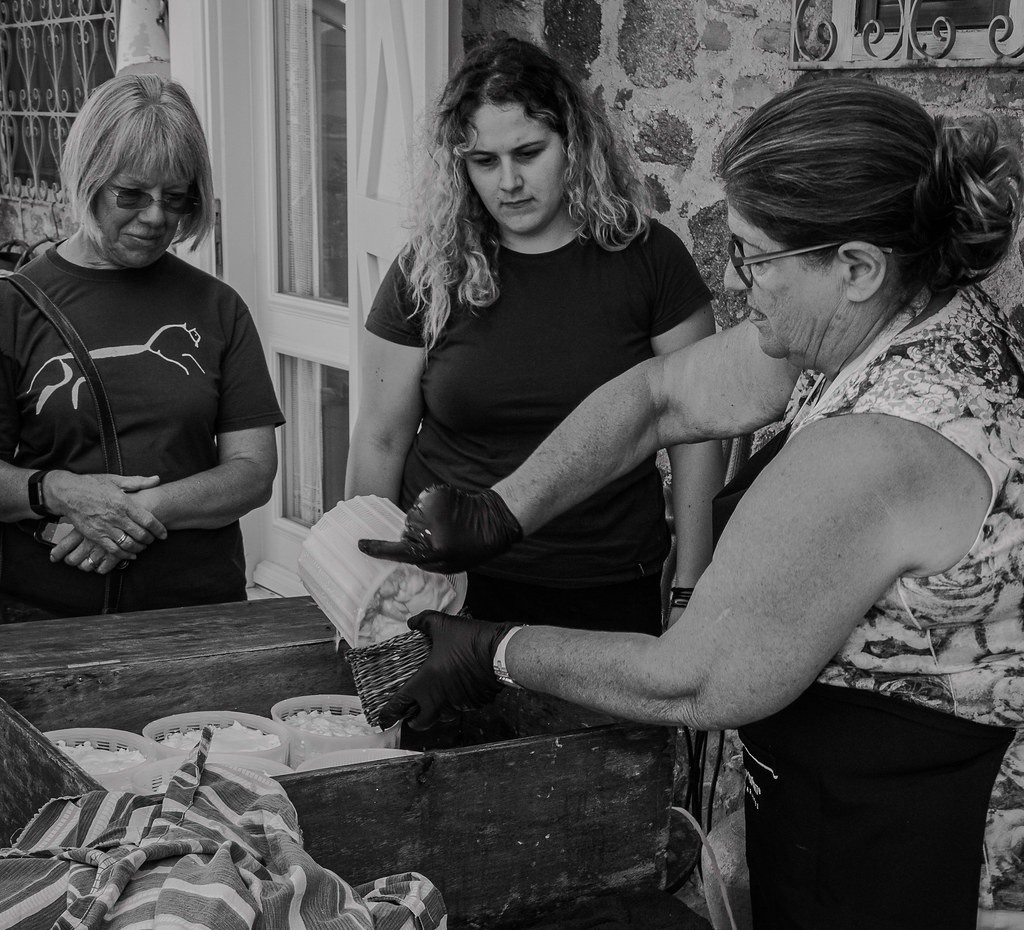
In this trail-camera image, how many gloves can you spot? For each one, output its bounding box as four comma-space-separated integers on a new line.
358, 484, 523, 577
375, 609, 516, 731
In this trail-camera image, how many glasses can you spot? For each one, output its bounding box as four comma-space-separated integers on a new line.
96, 183, 204, 217
728, 238, 895, 289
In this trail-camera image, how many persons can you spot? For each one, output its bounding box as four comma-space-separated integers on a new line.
357, 77, 1021, 930
342, 31, 720, 635
0, 74, 287, 608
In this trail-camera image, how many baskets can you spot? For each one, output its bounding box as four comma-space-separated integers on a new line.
346, 613, 481, 724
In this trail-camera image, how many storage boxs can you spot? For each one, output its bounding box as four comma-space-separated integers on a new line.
0, 618, 678, 927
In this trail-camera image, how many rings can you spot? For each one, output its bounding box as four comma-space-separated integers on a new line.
116, 532, 127, 547
88, 556, 96, 568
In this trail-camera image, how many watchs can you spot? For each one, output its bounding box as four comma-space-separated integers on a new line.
27, 469, 61, 523
490, 624, 526, 696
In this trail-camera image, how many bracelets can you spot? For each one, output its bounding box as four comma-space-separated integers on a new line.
670, 588, 696, 609
